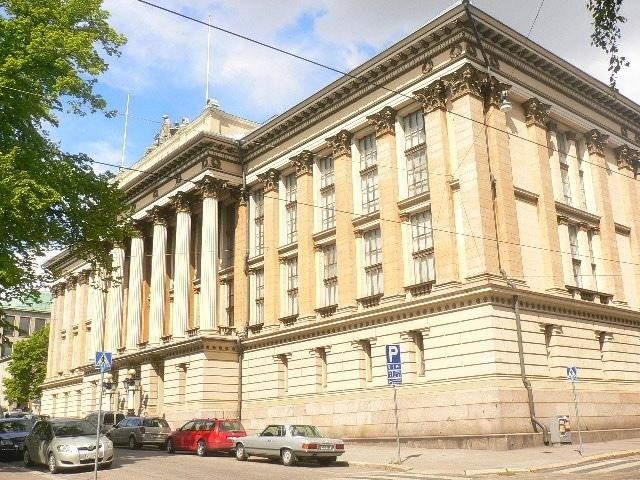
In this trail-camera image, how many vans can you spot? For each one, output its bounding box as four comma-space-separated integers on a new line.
82, 411, 126, 434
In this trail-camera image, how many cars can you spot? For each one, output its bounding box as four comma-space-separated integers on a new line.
0, 417, 34, 462
22, 414, 50, 428
165, 417, 247, 457
7, 413, 26, 418
104, 416, 171, 450
233, 423, 345, 466
22, 416, 115, 473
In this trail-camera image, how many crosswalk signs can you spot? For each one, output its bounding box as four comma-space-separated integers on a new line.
566, 366, 577, 380
95, 352, 112, 371
